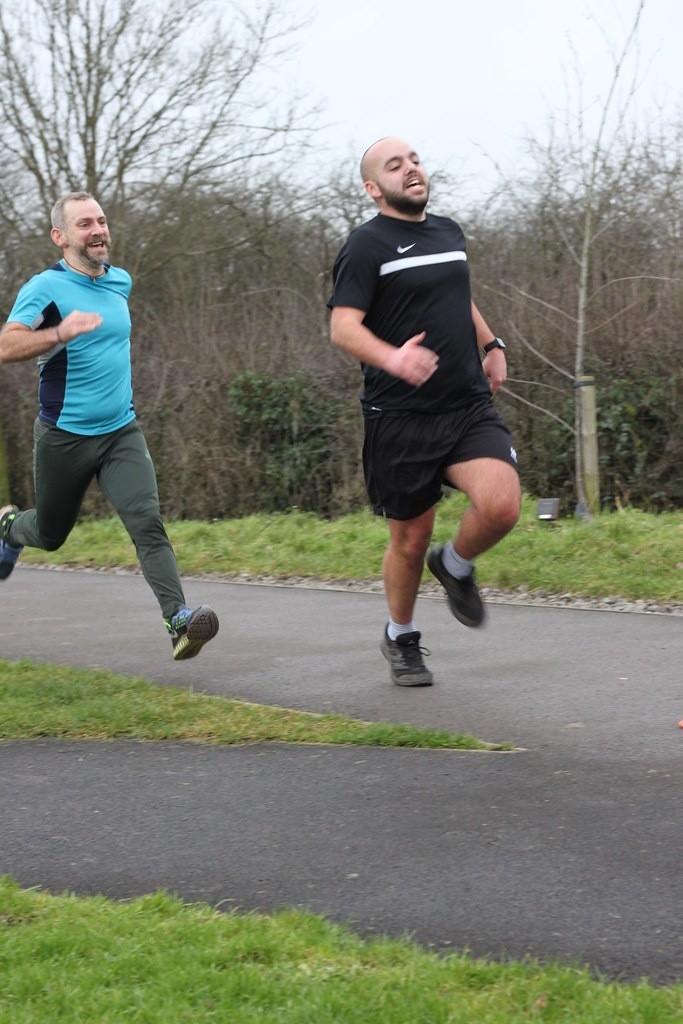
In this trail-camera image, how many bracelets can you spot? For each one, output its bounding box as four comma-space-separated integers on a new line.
57, 325, 63, 345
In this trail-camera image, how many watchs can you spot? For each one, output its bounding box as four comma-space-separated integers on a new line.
483, 338, 506, 355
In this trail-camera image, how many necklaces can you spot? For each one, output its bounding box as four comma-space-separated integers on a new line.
64, 257, 104, 283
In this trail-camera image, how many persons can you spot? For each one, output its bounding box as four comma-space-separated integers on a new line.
0, 191, 219, 662
325, 137, 524, 687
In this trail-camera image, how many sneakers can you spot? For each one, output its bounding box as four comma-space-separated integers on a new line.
165, 604, 219, 661
427, 544, 484, 626
380, 622, 434, 686
0, 504, 25, 579
